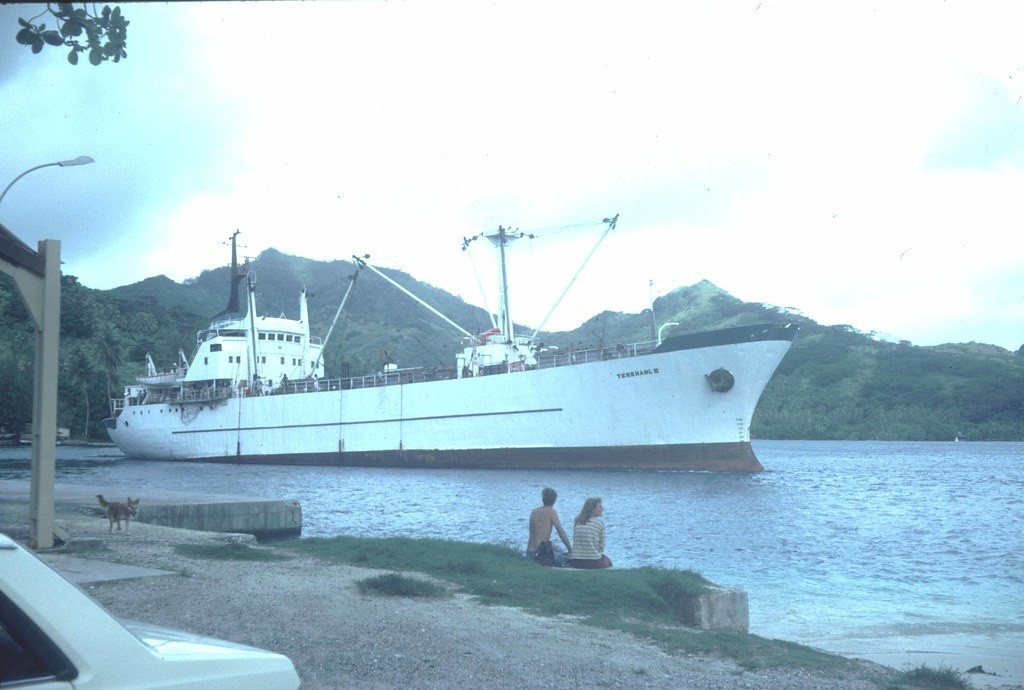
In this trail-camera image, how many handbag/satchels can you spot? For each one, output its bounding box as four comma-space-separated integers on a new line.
534, 541, 560, 566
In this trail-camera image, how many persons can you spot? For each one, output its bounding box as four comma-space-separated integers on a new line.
570, 498, 614, 569
526, 488, 572, 568
137, 373, 339, 404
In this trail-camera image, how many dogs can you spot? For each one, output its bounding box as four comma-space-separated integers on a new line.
96, 495, 140, 532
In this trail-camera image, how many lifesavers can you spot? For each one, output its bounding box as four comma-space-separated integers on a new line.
482, 328, 501, 346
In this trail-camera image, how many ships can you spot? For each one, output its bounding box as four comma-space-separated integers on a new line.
100, 210, 802, 474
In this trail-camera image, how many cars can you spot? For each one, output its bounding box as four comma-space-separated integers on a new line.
0, 536, 301, 690
